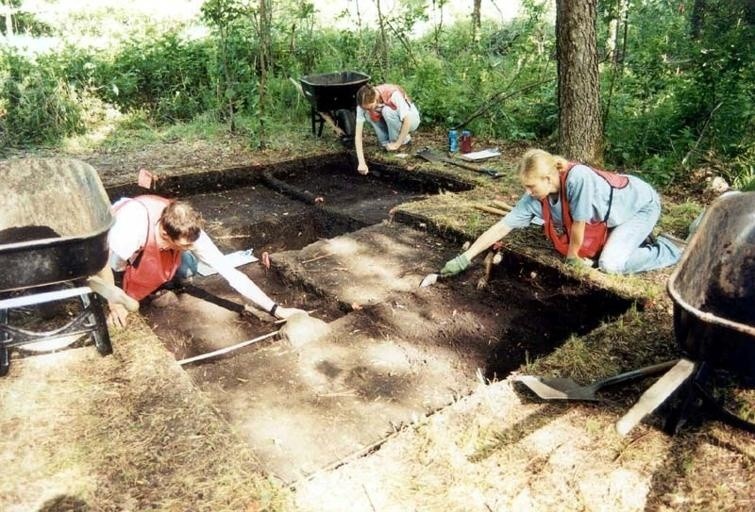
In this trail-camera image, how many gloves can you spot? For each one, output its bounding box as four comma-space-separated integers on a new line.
565, 257, 592, 281
440, 253, 471, 279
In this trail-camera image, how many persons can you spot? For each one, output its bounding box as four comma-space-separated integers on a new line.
97, 194, 309, 329
354, 83, 421, 175
439, 147, 681, 277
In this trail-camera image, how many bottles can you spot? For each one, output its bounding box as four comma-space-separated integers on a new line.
448, 127, 459, 152
461, 130, 472, 152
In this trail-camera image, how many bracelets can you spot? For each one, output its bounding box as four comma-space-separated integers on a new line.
270, 304, 278, 315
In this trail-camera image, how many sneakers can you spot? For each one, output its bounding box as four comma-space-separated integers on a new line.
661, 232, 689, 251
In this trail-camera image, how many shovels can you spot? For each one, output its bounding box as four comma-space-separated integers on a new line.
517, 356, 693, 405
415, 146, 499, 178
420, 271, 450, 289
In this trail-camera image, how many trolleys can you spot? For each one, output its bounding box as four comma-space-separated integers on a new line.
611, 190, 754, 435
0, 154, 118, 373
298, 71, 372, 149
